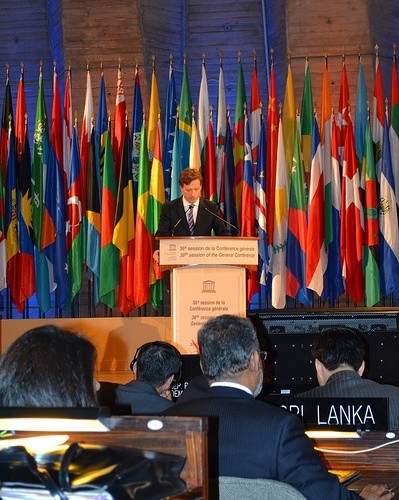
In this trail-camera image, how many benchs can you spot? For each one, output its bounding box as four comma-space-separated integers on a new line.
0, 407, 399, 500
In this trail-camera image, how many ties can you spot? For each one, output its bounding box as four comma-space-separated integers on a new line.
188, 205, 195, 235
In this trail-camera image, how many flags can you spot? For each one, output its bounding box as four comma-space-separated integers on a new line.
0, 58, 399, 318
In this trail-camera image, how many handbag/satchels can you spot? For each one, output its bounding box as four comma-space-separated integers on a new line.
0, 441, 187, 500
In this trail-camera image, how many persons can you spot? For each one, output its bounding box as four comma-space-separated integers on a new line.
153, 168, 230, 266
0, 324, 100, 407
294, 326, 399, 430
155, 314, 395, 500
96, 341, 182, 416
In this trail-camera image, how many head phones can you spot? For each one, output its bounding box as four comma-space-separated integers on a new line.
129, 340, 184, 388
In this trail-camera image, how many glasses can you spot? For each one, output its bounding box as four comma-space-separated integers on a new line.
254, 349, 267, 361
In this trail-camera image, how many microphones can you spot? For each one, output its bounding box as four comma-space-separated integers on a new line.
201, 205, 241, 236
172, 205, 194, 236
